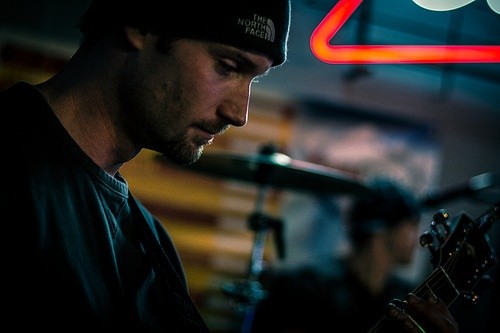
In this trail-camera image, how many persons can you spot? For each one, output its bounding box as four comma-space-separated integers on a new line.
0, 0, 293, 333
286, 182, 424, 333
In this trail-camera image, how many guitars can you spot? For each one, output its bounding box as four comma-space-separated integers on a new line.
369, 199, 500, 333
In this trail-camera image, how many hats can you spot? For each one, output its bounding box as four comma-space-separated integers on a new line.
78, 0, 293, 68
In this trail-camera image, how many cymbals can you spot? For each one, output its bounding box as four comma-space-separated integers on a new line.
154, 150, 374, 196
